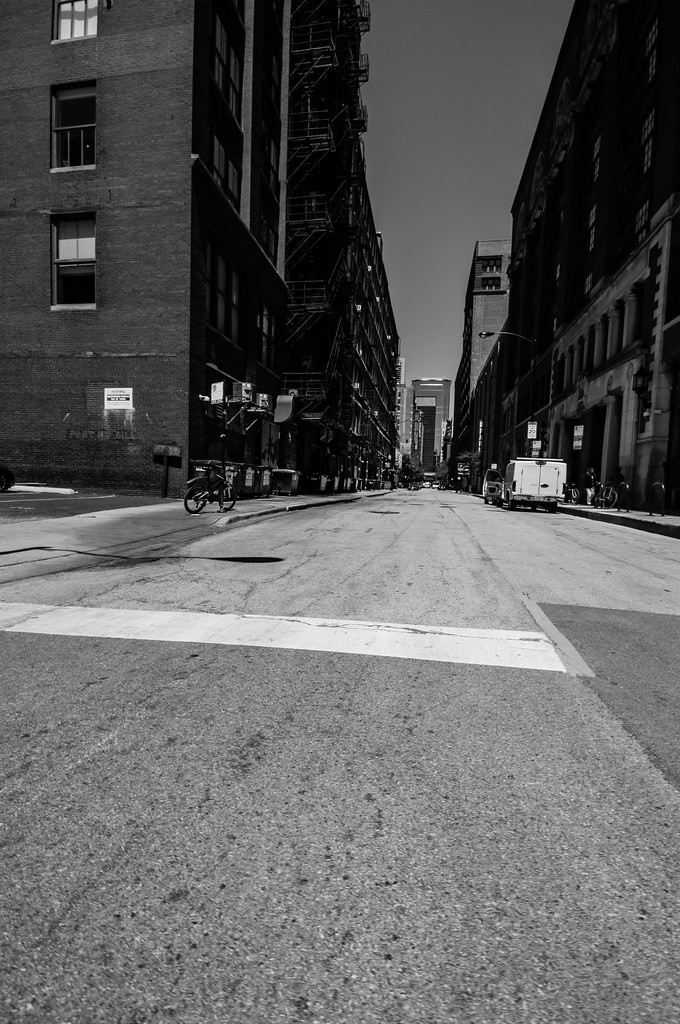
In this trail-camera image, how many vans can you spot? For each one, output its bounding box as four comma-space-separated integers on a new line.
483, 458, 567, 511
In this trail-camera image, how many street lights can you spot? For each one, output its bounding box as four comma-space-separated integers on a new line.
624, 365, 650, 511
478, 330, 537, 461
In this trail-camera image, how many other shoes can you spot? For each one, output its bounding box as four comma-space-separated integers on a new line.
587, 502, 592, 505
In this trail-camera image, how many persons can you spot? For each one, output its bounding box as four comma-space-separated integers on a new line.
609, 466, 625, 489
584, 468, 596, 506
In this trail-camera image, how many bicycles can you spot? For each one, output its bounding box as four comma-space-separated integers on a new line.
183, 464, 238, 513
594, 481, 617, 509
